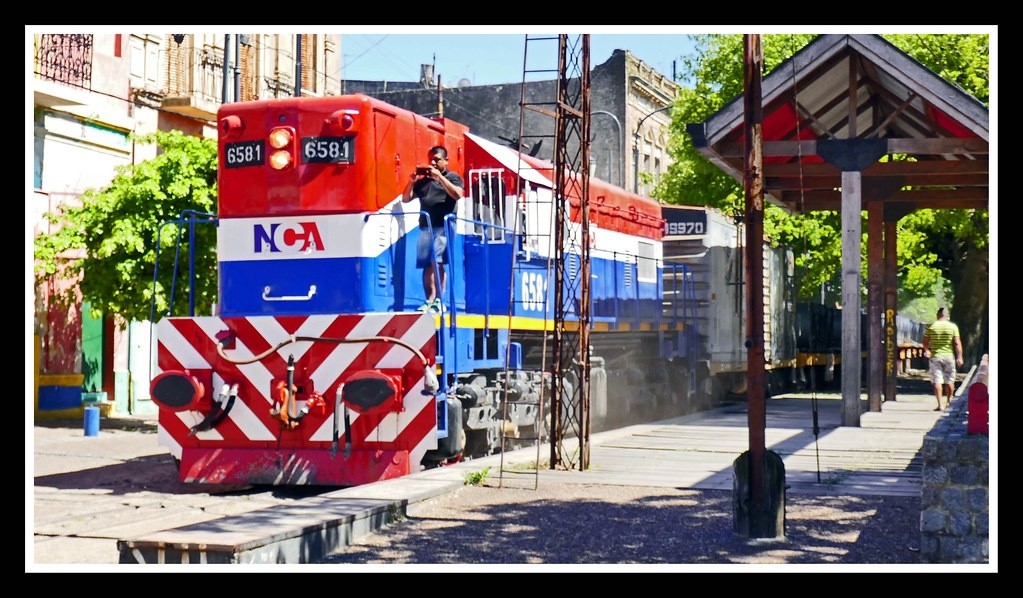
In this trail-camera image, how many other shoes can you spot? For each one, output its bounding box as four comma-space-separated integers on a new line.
944, 402, 951, 408
934, 407, 942, 411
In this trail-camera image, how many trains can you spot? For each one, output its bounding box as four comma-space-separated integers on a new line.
150, 94, 933, 489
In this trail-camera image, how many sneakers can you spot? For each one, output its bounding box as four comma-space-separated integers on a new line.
415, 299, 433, 313
428, 297, 447, 314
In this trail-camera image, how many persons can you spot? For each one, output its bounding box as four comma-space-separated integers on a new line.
402, 146, 464, 313
923, 308, 964, 411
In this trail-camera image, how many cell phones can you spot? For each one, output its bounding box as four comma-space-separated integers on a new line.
416, 168, 431, 176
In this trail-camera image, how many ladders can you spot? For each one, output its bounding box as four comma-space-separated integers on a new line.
499, 35, 561, 490
